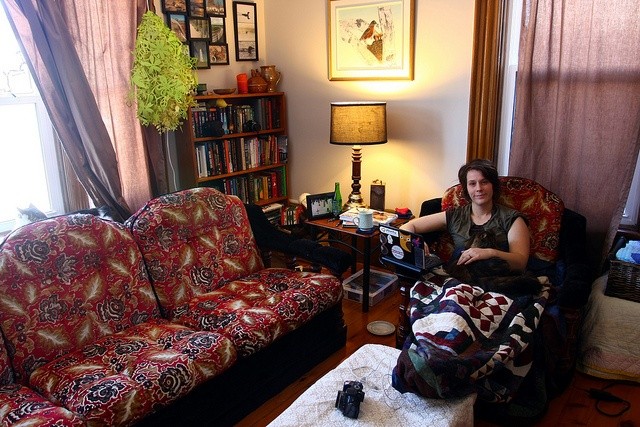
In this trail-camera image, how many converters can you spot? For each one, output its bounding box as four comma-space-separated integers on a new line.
589, 387, 625, 403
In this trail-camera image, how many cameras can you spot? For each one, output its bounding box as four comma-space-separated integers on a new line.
335, 381, 365, 419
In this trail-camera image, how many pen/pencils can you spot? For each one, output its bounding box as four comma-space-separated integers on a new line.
336, 220, 343, 227
356, 204, 372, 213
327, 217, 336, 221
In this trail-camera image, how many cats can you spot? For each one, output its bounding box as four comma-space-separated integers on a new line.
16, 204, 117, 229
447, 228, 538, 296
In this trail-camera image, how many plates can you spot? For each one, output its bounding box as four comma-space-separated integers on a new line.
365, 320, 395, 336
213, 88, 235, 94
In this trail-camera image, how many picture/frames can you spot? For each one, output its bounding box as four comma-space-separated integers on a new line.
233, 1, 258, 61
188, 17, 211, 40
307, 192, 335, 221
210, 16, 226, 43
205, 0, 226, 16
188, 1, 205, 17
209, 44, 229, 65
162, 0, 187, 13
167, 14, 188, 43
328, 0, 414, 81
189, 41, 210, 70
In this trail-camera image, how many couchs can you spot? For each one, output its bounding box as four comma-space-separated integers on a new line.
394, 176, 587, 378
0, 186, 353, 427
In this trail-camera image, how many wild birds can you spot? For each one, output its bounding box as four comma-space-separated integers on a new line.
358, 20, 379, 44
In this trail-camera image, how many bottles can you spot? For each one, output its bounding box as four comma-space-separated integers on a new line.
332, 182, 343, 218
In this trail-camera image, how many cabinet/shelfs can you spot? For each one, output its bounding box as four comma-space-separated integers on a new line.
174, 92, 291, 225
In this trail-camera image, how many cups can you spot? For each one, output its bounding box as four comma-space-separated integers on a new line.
353, 213, 373, 233
197, 84, 207, 95
237, 73, 249, 94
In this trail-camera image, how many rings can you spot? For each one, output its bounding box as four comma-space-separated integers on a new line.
468, 253, 473, 260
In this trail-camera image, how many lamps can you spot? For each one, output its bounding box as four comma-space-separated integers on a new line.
329, 102, 387, 211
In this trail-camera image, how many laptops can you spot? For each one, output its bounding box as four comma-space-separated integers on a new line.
378, 224, 444, 274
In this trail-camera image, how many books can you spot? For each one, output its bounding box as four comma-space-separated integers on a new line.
263, 204, 283, 225
191, 101, 253, 138
339, 205, 399, 228
256, 97, 282, 130
211, 167, 289, 204
196, 138, 287, 179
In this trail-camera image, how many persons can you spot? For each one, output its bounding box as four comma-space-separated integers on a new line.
399, 159, 531, 272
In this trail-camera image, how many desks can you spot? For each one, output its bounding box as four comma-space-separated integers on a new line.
305, 206, 415, 313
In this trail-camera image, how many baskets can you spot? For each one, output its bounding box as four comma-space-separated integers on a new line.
605, 237, 640, 303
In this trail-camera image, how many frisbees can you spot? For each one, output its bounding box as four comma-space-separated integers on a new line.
367, 321, 396, 336
